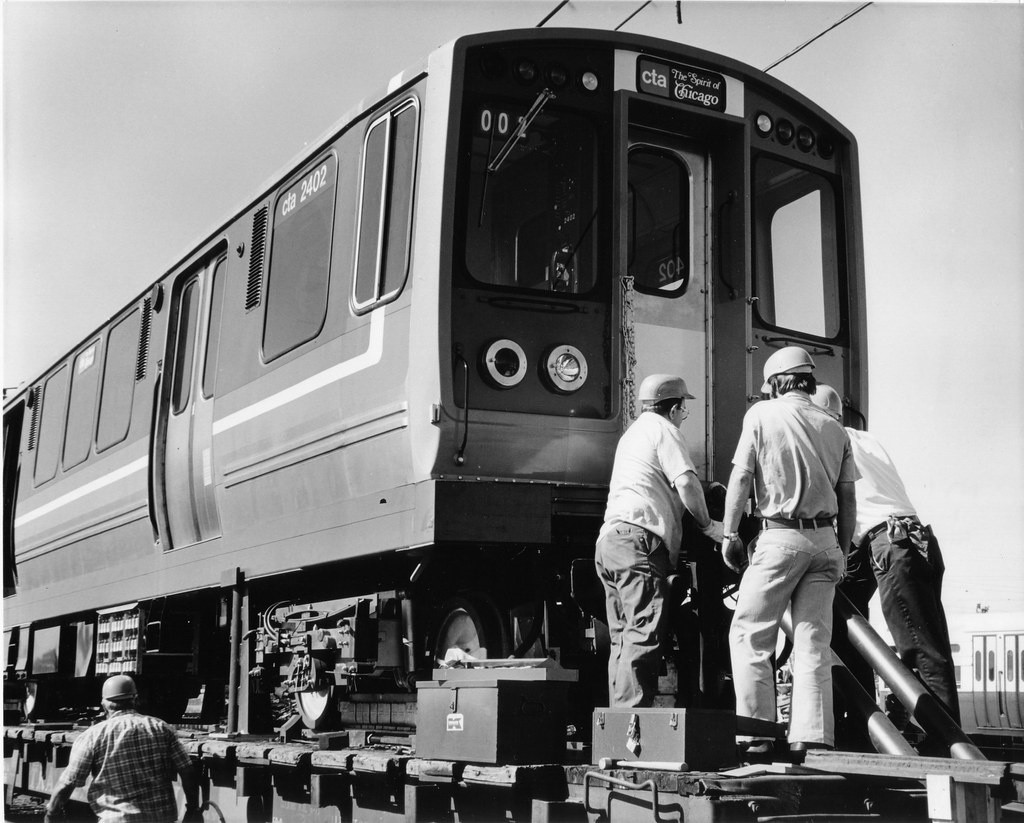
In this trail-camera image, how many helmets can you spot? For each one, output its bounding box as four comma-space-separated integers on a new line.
638, 373, 696, 405
760, 346, 816, 394
103, 674, 139, 700
808, 384, 843, 420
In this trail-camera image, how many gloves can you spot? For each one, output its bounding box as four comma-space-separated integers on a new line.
697, 519, 724, 543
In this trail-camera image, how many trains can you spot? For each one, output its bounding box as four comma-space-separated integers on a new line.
3, 29, 868, 747
873, 611, 1024, 762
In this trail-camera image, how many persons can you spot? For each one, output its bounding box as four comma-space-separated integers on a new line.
592, 373, 725, 708
805, 384, 966, 758
719, 345, 850, 763
43, 674, 205, 823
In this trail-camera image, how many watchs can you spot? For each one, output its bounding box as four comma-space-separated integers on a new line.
722, 532, 739, 542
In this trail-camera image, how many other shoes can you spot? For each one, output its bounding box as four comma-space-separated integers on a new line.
740, 740, 784, 765
789, 743, 825, 763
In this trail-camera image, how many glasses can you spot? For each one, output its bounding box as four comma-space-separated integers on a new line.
678, 406, 690, 421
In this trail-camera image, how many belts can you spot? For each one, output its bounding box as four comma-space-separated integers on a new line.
851, 515, 920, 561
757, 518, 833, 533
